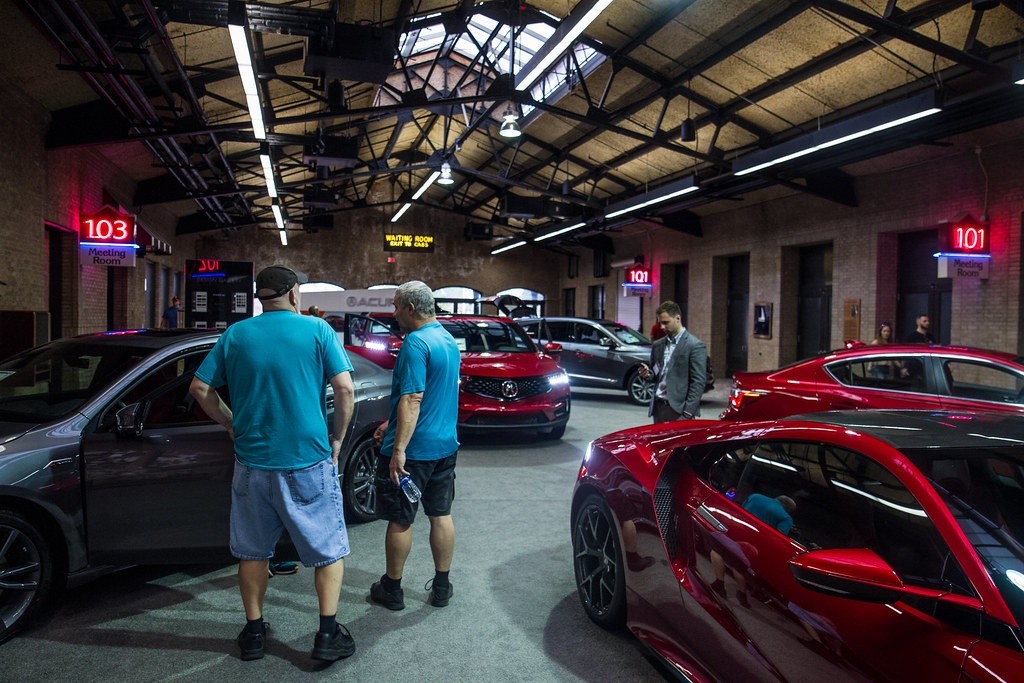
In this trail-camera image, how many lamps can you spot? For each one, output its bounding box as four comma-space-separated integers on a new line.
561, 150, 574, 196
438, 159, 454, 184
328, 78, 345, 112
732, 63, 944, 175
533, 217, 588, 242
411, 168, 441, 200
226, 14, 267, 141
259, 149, 278, 198
499, 98, 521, 137
604, 123, 702, 219
390, 200, 412, 222
316, 165, 329, 180
309, 126, 324, 154
271, 200, 287, 245
681, 78, 696, 142
491, 235, 531, 255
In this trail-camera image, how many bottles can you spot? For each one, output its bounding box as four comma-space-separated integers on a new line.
398, 472, 421, 503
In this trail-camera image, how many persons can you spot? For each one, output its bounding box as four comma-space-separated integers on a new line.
638, 301, 707, 424
161, 296, 182, 329
370, 280, 461, 611
189, 265, 357, 662
309, 306, 319, 316
350, 312, 371, 345
867, 315, 934, 381
615, 458, 842, 660
650, 324, 666, 342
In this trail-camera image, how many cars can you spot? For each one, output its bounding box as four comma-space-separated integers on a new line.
0, 330, 397, 644
720, 339, 1024, 416
349, 312, 405, 354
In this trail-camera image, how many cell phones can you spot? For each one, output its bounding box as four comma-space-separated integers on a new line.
641, 364, 652, 374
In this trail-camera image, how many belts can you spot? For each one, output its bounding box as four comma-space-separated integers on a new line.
654, 397, 669, 407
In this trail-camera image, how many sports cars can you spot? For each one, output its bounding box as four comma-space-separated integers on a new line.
570, 408, 1024, 683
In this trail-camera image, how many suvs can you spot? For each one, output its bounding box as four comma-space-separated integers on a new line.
343, 312, 571, 440
475, 289, 716, 405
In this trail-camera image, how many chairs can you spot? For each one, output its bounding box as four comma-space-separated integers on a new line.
581, 326, 594, 344
90, 355, 231, 428
903, 362, 955, 395
916, 476, 1015, 577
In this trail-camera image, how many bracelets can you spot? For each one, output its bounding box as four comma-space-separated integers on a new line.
683, 413, 691, 418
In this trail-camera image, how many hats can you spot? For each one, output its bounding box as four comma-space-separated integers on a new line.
254, 265, 301, 300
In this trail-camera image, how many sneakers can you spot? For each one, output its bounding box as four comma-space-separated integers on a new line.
736, 591, 751, 609
270, 559, 298, 577
628, 555, 655, 572
370, 582, 406, 611
310, 619, 356, 660
236, 618, 271, 660
424, 577, 454, 606
710, 578, 727, 598
